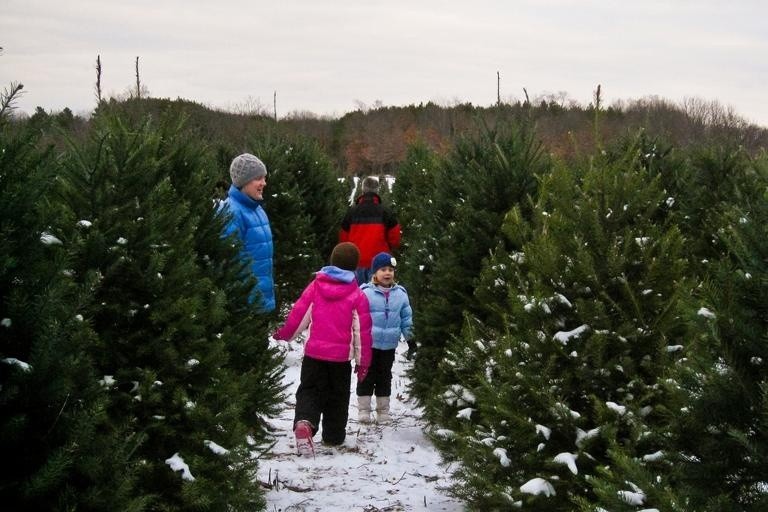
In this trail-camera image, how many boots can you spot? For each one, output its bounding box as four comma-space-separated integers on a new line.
295, 419, 315, 461
358, 395, 371, 424
376, 396, 392, 423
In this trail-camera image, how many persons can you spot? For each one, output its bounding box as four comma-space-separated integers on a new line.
273, 241, 373, 448
359, 252, 418, 428
338, 177, 402, 284
220, 153, 290, 440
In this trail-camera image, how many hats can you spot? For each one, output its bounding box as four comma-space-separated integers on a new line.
330, 242, 358, 270
363, 177, 378, 192
372, 252, 397, 273
230, 153, 267, 187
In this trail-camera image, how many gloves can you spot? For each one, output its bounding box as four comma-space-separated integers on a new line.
273, 332, 284, 340
354, 365, 368, 383
407, 340, 417, 361
